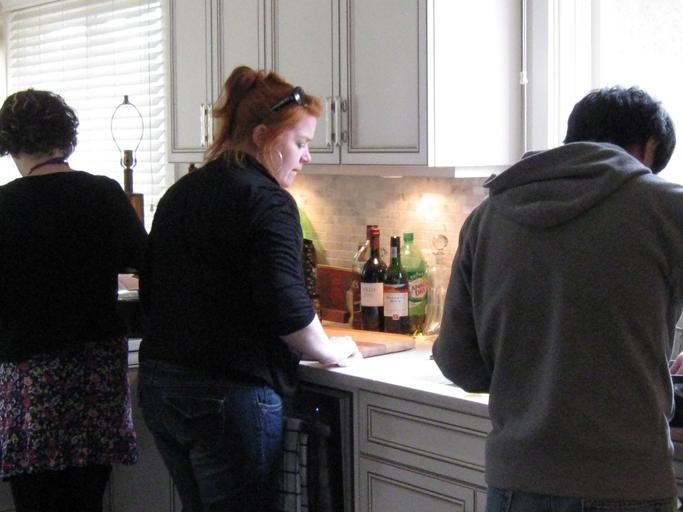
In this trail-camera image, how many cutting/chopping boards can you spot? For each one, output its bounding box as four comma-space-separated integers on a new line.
298, 321, 416, 371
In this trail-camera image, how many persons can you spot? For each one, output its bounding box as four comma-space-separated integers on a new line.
431, 80, 683, 512
0, 86, 151, 512
134, 65, 358, 512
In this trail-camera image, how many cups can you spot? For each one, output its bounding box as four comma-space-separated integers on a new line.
668, 373, 682, 428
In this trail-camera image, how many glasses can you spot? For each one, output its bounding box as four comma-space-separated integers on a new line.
272, 86, 306, 109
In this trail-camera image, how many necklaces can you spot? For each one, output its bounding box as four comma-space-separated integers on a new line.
25, 157, 68, 176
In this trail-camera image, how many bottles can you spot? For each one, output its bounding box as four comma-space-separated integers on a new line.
358, 228, 389, 332
382, 235, 409, 335
350, 223, 389, 331
398, 232, 427, 335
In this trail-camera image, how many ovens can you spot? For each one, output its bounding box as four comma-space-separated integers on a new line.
271, 382, 344, 512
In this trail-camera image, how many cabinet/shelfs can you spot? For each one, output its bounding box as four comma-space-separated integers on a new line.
266, 1, 526, 180
104, 350, 185, 511
355, 387, 494, 511
161, 1, 266, 164
